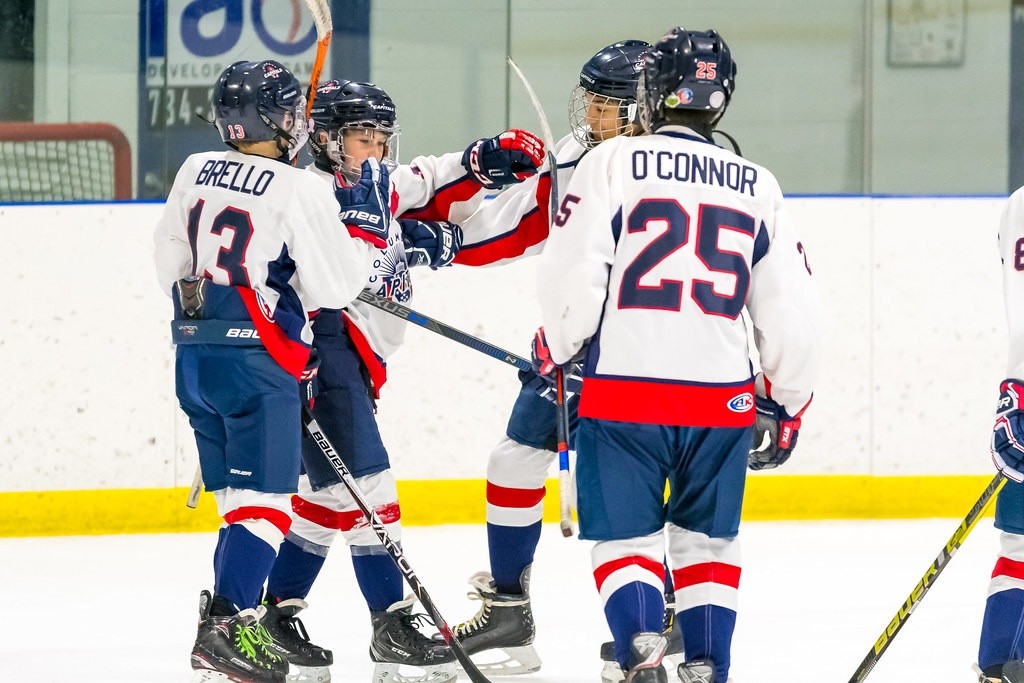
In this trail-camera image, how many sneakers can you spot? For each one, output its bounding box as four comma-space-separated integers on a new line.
190, 590, 291, 683
369, 592, 460, 683
253, 595, 335, 683
431, 562, 542, 678
599, 607, 686, 683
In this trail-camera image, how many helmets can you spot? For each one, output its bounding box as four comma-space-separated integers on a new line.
211, 59, 307, 162
306, 77, 401, 176
566, 38, 656, 153
635, 25, 738, 135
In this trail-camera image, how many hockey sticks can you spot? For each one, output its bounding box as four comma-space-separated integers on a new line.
848, 470, 1011, 682
187, 1, 333, 512
291, 395, 493, 683
510, 58, 575, 541
355, 292, 586, 395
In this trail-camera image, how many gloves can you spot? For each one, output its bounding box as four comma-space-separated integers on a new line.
331, 156, 400, 250
989, 410, 1024, 481
517, 325, 580, 407
746, 395, 803, 471
399, 217, 464, 271
460, 127, 545, 190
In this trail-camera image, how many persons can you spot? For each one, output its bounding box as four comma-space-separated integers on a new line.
973, 184, 1024, 683
262, 78, 546, 683
397, 38, 686, 677
529, 22, 821, 683
151, 59, 371, 683
